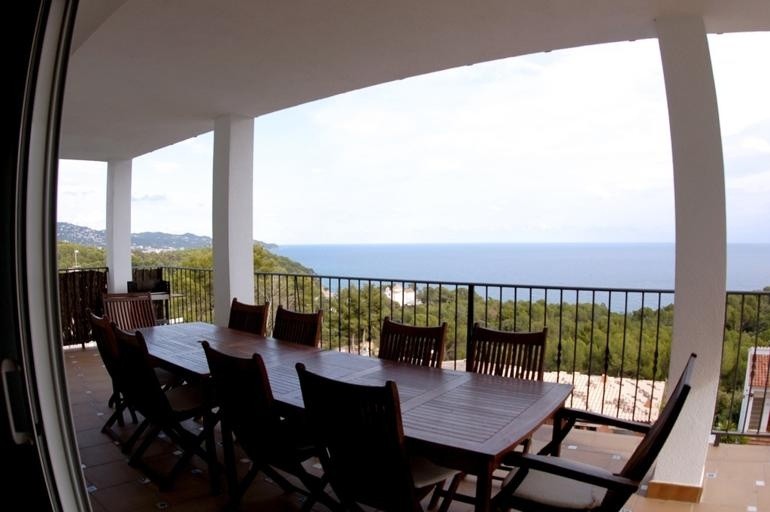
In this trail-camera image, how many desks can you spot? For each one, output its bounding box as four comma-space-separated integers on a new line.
124, 322, 573, 512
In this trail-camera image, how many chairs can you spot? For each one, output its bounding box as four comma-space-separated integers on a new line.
437, 321, 547, 510
200, 341, 343, 511
378, 315, 448, 368
228, 297, 270, 336
294, 361, 466, 512
491, 352, 698, 512
272, 305, 323, 348
83, 305, 222, 495
100, 290, 158, 408
